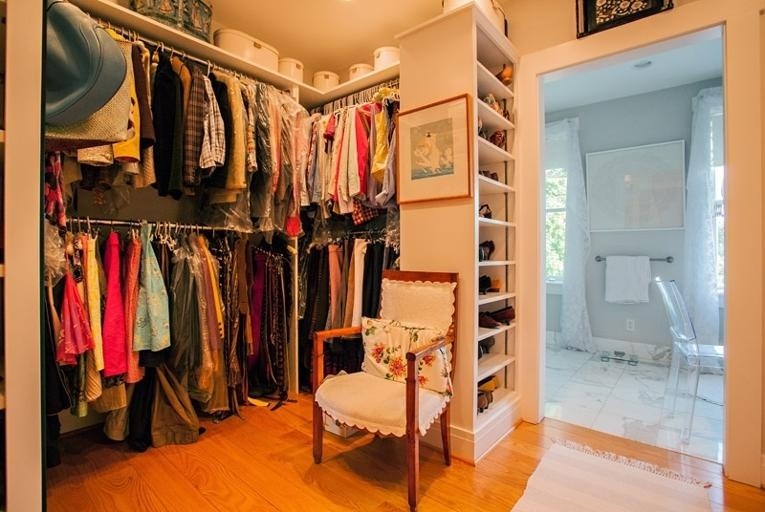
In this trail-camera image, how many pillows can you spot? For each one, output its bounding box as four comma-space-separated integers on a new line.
361, 316, 453, 397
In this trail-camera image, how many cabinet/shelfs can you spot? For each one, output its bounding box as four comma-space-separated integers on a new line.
0, 126, 5, 413
395, 1, 522, 466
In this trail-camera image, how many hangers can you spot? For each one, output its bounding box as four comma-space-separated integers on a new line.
96, 18, 262, 84
328, 228, 388, 246
60, 214, 288, 265
306, 79, 399, 115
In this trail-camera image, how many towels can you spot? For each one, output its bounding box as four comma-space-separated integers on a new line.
604, 255, 652, 303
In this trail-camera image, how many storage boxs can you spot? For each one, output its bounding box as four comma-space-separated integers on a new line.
348, 64, 374, 80
278, 57, 304, 82
119, 0, 214, 42
442, 0, 509, 38
373, 46, 400, 72
213, 28, 280, 74
312, 71, 340, 93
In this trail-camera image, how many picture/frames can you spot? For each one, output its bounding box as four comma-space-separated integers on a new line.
395, 93, 474, 205
585, 139, 686, 232
575, 0, 674, 39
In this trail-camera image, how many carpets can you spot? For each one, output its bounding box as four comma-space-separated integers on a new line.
509, 438, 710, 511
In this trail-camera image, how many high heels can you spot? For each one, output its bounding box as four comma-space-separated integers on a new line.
479, 67, 515, 412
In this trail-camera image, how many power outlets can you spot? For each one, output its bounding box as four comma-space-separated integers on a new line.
626, 319, 635, 333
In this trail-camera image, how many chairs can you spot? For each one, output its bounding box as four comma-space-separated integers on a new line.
655, 275, 724, 446
312, 269, 461, 512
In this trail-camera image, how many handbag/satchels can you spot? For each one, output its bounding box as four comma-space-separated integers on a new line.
46, 1, 133, 153
